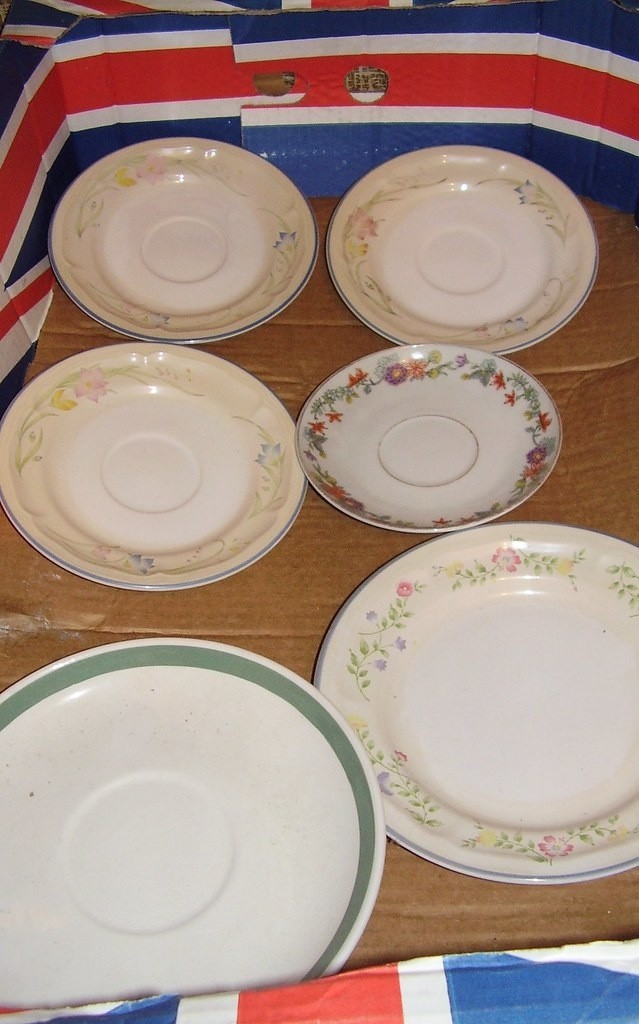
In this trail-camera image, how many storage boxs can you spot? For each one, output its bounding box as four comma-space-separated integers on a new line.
1, 2, 639, 1021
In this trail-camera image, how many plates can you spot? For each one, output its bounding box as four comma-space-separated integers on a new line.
0, 341, 310, 592
0, 634, 387, 1006
46, 136, 320, 342
314, 522, 639, 892
292, 343, 563, 533
324, 138, 601, 365
